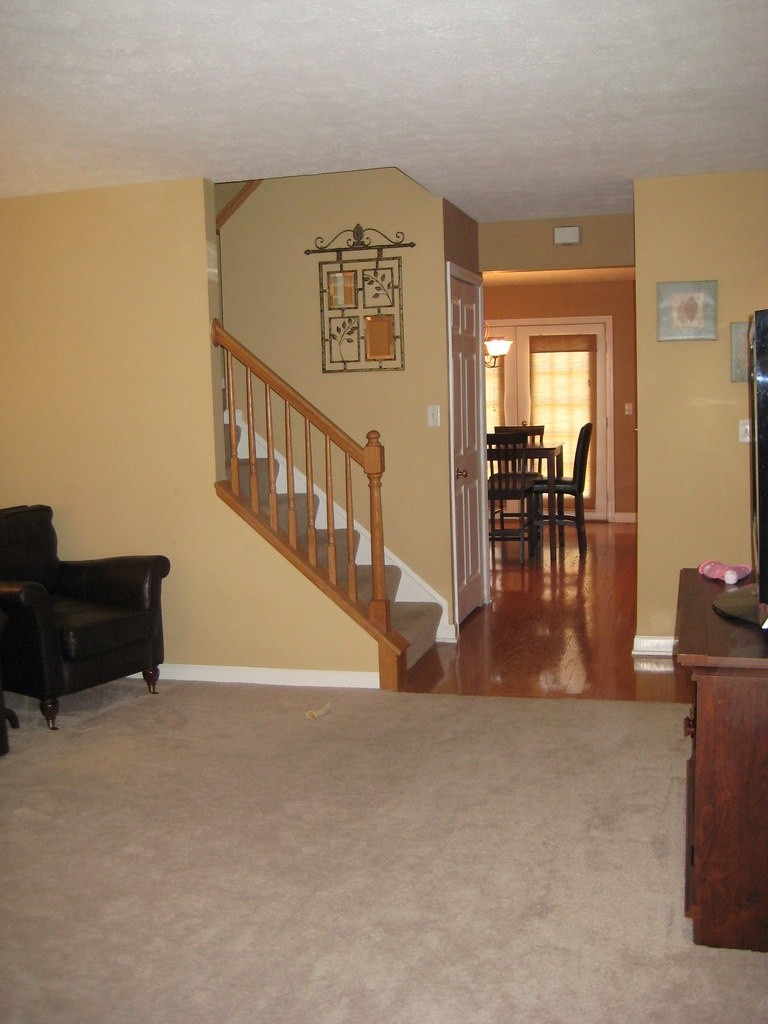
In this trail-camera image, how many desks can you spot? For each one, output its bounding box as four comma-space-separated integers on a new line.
486, 440, 566, 558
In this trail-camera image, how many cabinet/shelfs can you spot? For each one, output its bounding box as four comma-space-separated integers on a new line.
671, 567, 768, 954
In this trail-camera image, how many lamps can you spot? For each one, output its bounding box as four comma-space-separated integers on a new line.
484, 320, 514, 368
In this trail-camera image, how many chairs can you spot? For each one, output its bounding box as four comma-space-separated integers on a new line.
0, 503, 172, 730
485, 431, 541, 562
491, 424, 547, 543
529, 422, 594, 555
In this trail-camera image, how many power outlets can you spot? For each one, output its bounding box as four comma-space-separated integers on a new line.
625, 402, 633, 416
